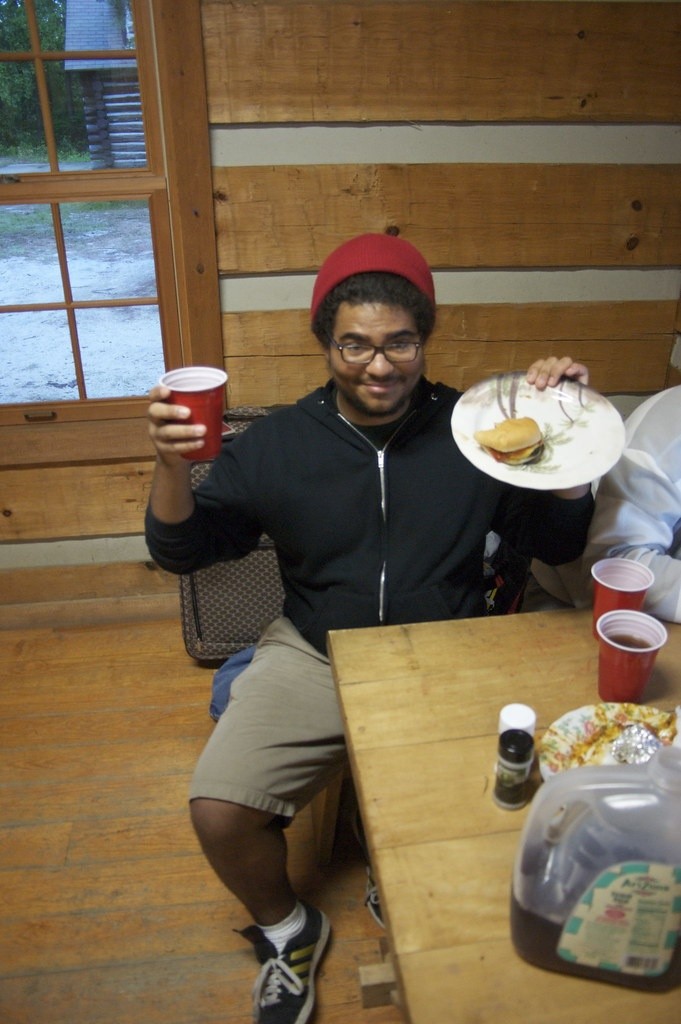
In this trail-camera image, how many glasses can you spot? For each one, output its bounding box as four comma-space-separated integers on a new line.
324, 327, 424, 365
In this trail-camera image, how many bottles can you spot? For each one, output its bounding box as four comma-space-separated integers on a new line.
493, 730, 534, 810
495, 704, 537, 773
510, 746, 681, 993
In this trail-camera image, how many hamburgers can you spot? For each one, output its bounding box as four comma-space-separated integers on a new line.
474, 416, 543, 464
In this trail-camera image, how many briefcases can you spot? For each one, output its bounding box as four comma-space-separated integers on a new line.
176, 405, 316, 662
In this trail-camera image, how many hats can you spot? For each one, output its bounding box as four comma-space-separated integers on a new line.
310, 233, 436, 342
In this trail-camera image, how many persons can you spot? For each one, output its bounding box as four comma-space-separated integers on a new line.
146, 236, 594, 1024
520, 383, 681, 625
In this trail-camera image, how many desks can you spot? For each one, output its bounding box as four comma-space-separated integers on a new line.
326, 605, 681, 1024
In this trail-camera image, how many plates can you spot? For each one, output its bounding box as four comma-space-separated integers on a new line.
452, 372, 628, 490
536, 703, 681, 782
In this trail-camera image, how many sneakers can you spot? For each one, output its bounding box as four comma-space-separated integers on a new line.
231, 899, 331, 1024
349, 803, 387, 930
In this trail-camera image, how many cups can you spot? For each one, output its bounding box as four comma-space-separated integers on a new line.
596, 609, 667, 703
160, 366, 229, 461
591, 559, 655, 641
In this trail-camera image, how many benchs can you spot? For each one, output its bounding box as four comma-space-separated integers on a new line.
176, 407, 352, 869
